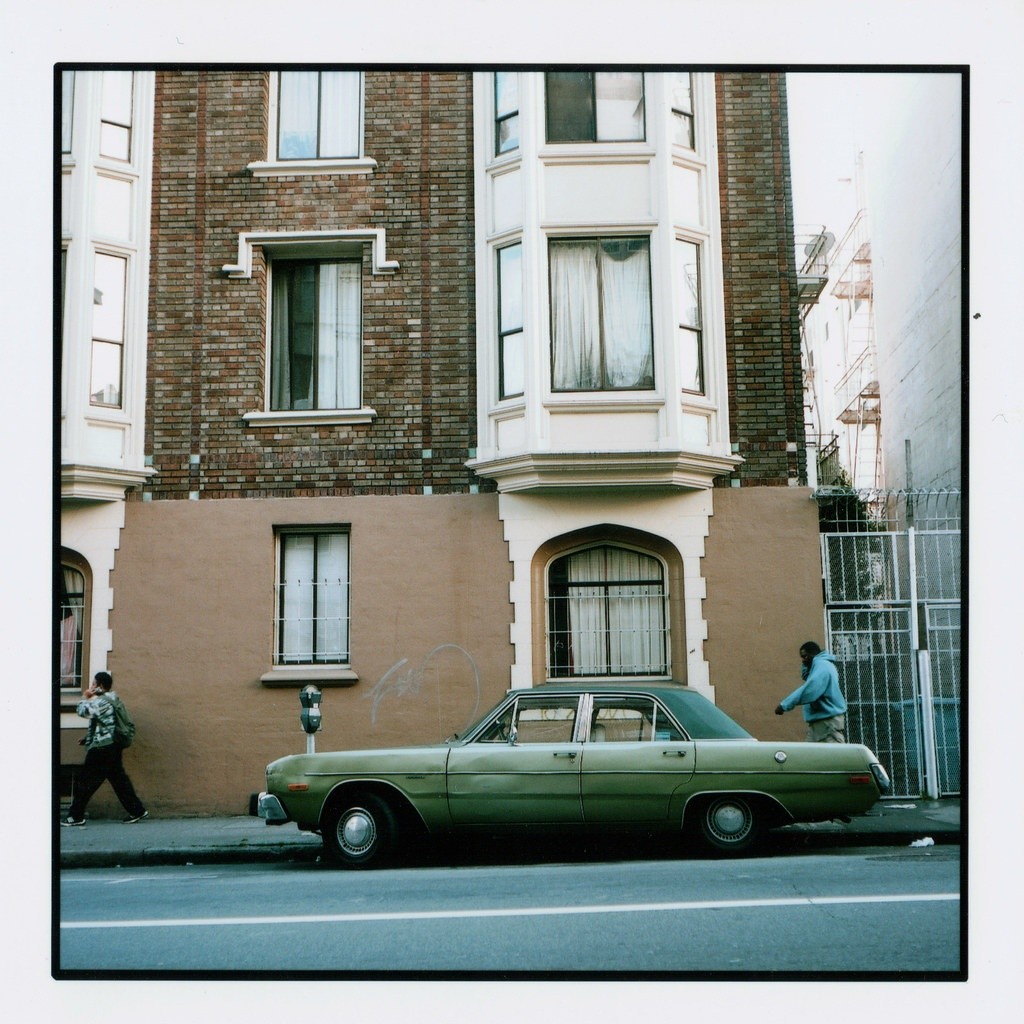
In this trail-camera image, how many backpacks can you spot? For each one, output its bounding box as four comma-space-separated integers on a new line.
97, 694, 135, 749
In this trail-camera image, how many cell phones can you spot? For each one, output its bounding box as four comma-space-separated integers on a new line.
93, 687, 102, 692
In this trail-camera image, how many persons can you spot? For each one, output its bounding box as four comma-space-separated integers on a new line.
774, 642, 848, 743
60, 672, 149, 827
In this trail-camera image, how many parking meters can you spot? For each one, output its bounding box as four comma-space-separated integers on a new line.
299, 685, 323, 754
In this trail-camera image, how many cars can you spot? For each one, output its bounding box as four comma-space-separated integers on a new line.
260, 686, 890, 870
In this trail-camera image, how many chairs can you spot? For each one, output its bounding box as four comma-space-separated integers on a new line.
594, 724, 606, 742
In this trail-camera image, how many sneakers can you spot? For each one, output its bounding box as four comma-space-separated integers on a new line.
122, 809, 149, 824
60, 816, 86, 827
